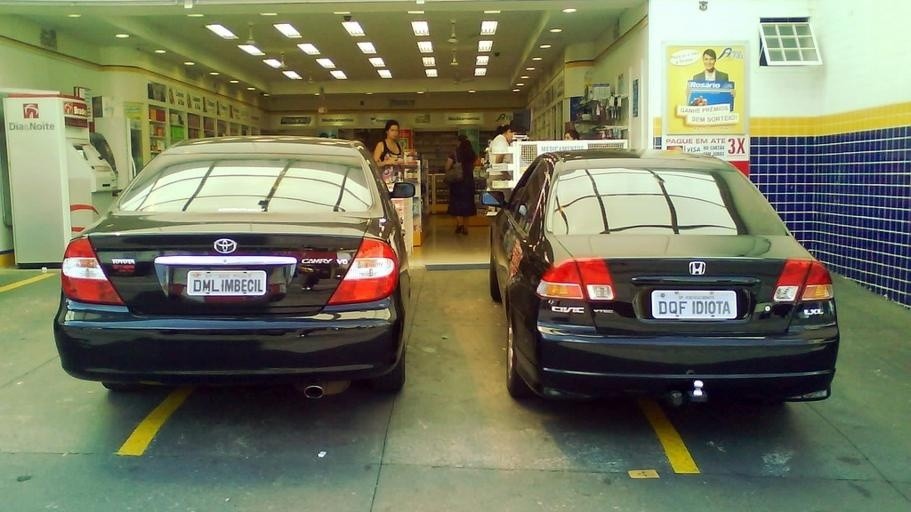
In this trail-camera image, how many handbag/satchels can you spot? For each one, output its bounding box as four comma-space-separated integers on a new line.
443, 165, 464, 186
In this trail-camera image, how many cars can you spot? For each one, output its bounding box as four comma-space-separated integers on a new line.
479, 148, 843, 407
54, 133, 416, 402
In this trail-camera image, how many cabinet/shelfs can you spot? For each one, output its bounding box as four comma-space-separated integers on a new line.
140, 98, 261, 169
427, 165, 496, 226
579, 94, 628, 131
403, 159, 429, 247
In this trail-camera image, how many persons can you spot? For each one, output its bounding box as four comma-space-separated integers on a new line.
444, 134, 479, 235
487, 125, 515, 201
563, 129, 581, 140
694, 51, 731, 79
373, 120, 403, 167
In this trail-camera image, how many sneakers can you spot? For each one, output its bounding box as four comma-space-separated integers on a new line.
455, 224, 468, 235
